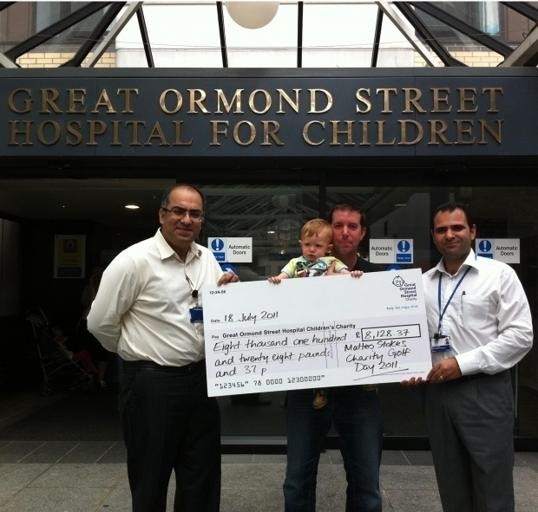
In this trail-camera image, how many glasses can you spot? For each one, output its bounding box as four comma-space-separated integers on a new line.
160, 207, 207, 223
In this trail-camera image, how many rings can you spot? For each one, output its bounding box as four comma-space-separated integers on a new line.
439, 375, 444, 381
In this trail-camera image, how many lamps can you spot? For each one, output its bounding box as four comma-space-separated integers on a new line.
224, 2, 279, 30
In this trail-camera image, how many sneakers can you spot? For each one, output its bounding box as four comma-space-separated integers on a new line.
312, 388, 336, 410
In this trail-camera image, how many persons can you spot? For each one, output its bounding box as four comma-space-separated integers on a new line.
85, 181, 241, 512
265, 217, 366, 409
398, 202, 534, 512
282, 202, 383, 512
